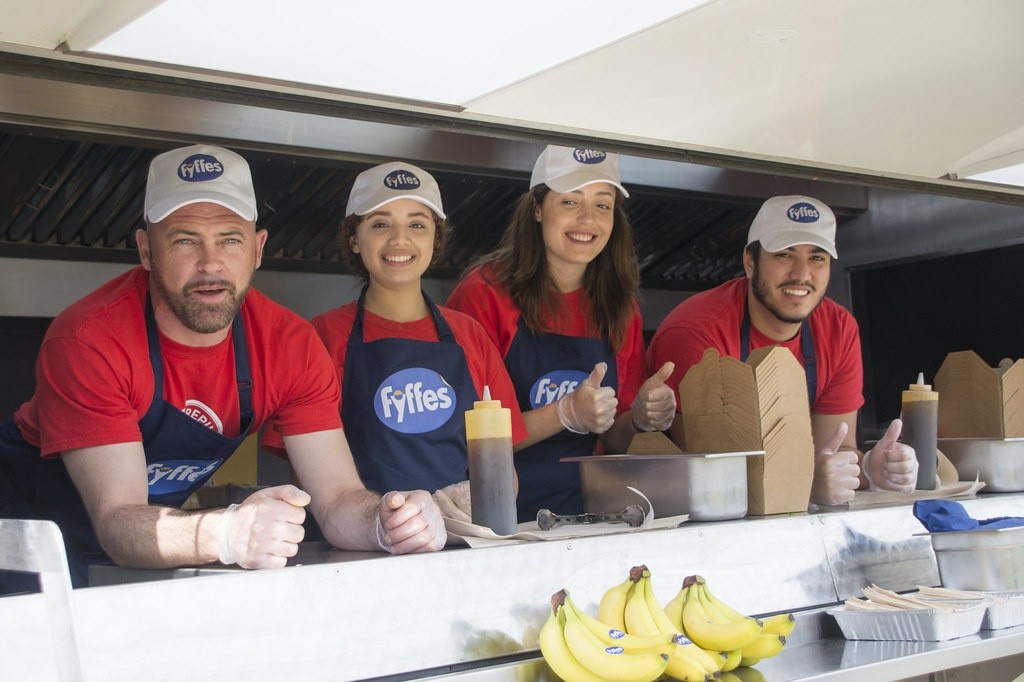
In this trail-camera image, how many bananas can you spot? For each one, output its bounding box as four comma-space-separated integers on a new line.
538, 562, 795, 681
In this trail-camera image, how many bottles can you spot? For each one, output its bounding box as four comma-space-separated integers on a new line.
465, 386, 518, 537
901, 373, 938, 490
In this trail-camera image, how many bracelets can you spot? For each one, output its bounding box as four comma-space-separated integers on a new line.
374, 506, 394, 554
216, 501, 241, 567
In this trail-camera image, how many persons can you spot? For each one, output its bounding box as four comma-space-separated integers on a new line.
260, 160, 532, 549
595, 193, 923, 516
0, 141, 448, 597
441, 143, 674, 536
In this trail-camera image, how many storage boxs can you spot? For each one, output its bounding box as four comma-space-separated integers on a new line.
557, 340, 1024, 644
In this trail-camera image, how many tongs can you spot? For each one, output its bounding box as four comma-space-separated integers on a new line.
536, 504, 645, 530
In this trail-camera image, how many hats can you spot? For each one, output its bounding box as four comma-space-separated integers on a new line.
529, 143, 631, 198
142, 143, 258, 225
345, 160, 447, 219
748, 193, 839, 260
